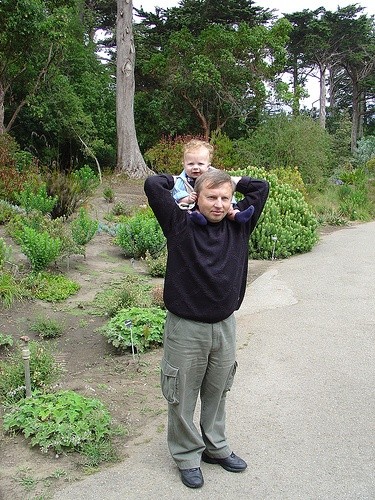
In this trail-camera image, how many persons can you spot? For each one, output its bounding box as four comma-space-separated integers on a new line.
144, 170, 270, 488
171, 139, 254, 225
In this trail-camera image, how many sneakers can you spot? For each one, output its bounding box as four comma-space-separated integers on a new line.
201, 447, 248, 473
178, 467, 204, 488
